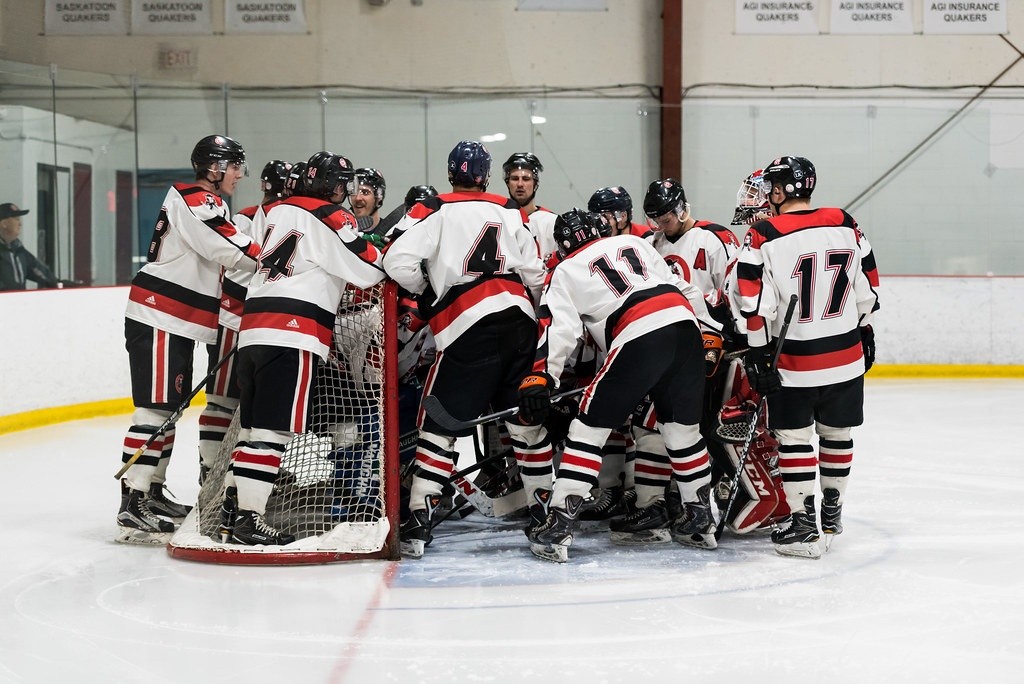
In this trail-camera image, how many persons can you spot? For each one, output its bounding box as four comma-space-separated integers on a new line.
0, 203, 59, 293
283, 151, 739, 545
197, 156, 298, 487
382, 140, 556, 559
712, 167, 793, 537
112, 132, 261, 546
735, 155, 880, 560
216, 150, 386, 548
517, 207, 719, 563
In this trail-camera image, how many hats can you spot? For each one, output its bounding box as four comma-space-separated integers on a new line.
0, 203, 29, 221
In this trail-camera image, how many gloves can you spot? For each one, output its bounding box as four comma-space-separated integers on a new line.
859, 325, 876, 372
702, 331, 723, 380
744, 348, 782, 396
516, 372, 555, 423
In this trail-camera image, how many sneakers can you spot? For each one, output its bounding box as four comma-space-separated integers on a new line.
216, 473, 242, 546
396, 511, 431, 560
608, 475, 733, 551
822, 487, 843, 552
232, 508, 296, 546
528, 495, 584, 563
521, 486, 552, 541
144, 481, 194, 525
580, 485, 631, 534
115, 479, 184, 546
770, 495, 820, 559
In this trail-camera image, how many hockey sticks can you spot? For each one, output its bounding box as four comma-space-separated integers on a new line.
114, 341, 238, 480
424, 438, 569, 545
712, 293, 799, 542
421, 385, 587, 431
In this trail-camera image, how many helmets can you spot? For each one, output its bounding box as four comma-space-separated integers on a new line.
357, 167, 386, 208
588, 186, 632, 222
260, 151, 355, 197
643, 177, 687, 219
447, 141, 491, 189
553, 207, 595, 257
502, 152, 543, 178
584, 208, 613, 239
731, 169, 776, 226
190, 134, 246, 173
404, 185, 438, 212
762, 156, 818, 199
